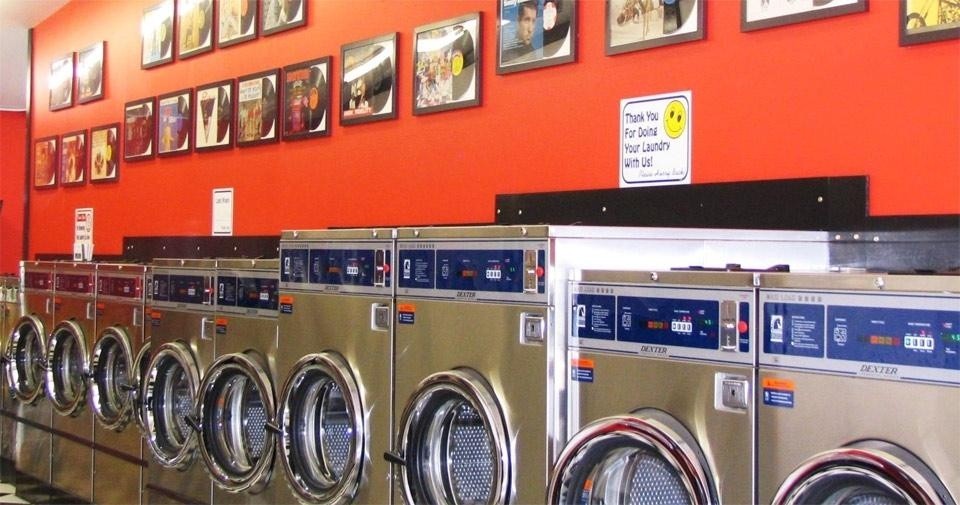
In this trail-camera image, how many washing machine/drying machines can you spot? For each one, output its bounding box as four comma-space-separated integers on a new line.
138, 262, 213, 505
266, 229, 392, 505
0, 261, 56, 489
545, 270, 756, 503
83, 264, 146, 504
120, 267, 152, 504
184, 258, 275, 504
33, 262, 98, 504
757, 270, 956, 504
392, 225, 830, 505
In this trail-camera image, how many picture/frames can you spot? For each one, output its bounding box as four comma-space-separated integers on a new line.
76, 40, 106, 105
604, 1, 708, 56
740, 0, 869, 33
194, 77, 236, 153
37, 88, 40, 89
261, 0, 307, 36
48, 52, 76, 112
33, 135, 59, 191
123, 95, 157, 163
89, 122, 121, 184
178, 0, 214, 60
216, 0, 258, 49
494, 0, 578, 74
280, 55, 333, 142
60, 129, 88, 187
235, 68, 281, 148
157, 87, 194, 158
899, 0, 960, 47
140, 0, 175, 71
412, 12, 482, 115
339, 32, 400, 126
